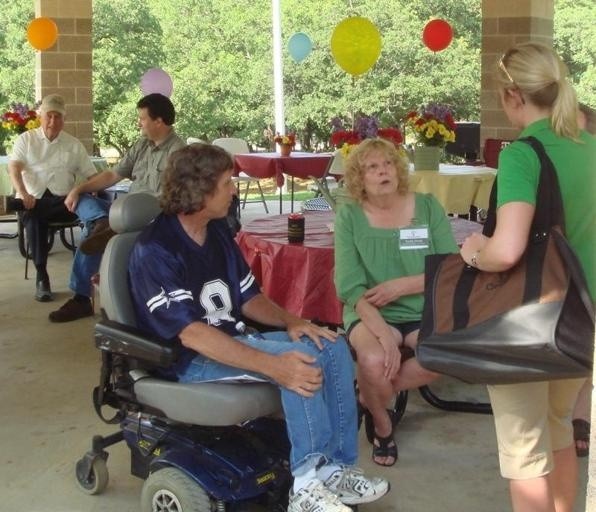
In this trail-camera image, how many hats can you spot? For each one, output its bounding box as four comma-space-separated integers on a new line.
41, 95, 66, 116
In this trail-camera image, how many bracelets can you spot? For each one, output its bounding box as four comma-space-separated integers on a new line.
471, 248, 481, 268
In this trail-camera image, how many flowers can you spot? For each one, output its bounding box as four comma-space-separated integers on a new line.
274, 129, 296, 145
1, 100, 42, 158
329, 111, 403, 159
405, 103, 457, 145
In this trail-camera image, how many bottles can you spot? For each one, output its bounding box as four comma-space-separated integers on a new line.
287, 212, 304, 244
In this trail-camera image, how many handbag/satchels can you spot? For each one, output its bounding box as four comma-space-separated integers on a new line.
415, 221, 594, 384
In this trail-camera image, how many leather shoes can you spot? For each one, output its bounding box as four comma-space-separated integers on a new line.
4, 196, 17, 212
34, 280, 52, 301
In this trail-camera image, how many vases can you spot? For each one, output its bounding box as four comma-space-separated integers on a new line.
279, 145, 291, 156
414, 146, 440, 170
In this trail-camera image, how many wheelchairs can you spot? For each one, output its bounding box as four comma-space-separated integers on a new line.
76, 192, 363, 512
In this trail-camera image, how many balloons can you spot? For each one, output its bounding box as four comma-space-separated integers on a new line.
421, 19, 453, 56
288, 32, 312, 67
26, 17, 58, 52
141, 69, 172, 101
331, 16, 382, 78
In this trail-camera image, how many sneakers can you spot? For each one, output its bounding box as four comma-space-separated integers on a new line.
323, 464, 390, 505
287, 477, 354, 512
49, 299, 92, 322
79, 217, 115, 256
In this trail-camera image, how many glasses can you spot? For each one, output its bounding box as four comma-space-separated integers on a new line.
498, 49, 526, 104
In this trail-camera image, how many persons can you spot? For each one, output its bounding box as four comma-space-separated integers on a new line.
1, 92, 103, 303
331, 138, 459, 467
48, 94, 188, 322
460, 43, 595, 511
125, 142, 392, 511
570, 374, 593, 459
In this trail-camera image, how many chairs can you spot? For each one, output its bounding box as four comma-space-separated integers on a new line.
210, 137, 269, 214
186, 137, 207, 146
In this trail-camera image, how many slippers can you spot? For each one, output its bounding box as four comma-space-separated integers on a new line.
573, 418, 591, 457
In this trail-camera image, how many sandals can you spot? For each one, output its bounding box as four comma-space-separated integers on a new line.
373, 427, 397, 466
354, 379, 366, 431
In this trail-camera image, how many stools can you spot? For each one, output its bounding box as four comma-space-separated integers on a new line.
349, 344, 493, 444
17, 213, 84, 281
